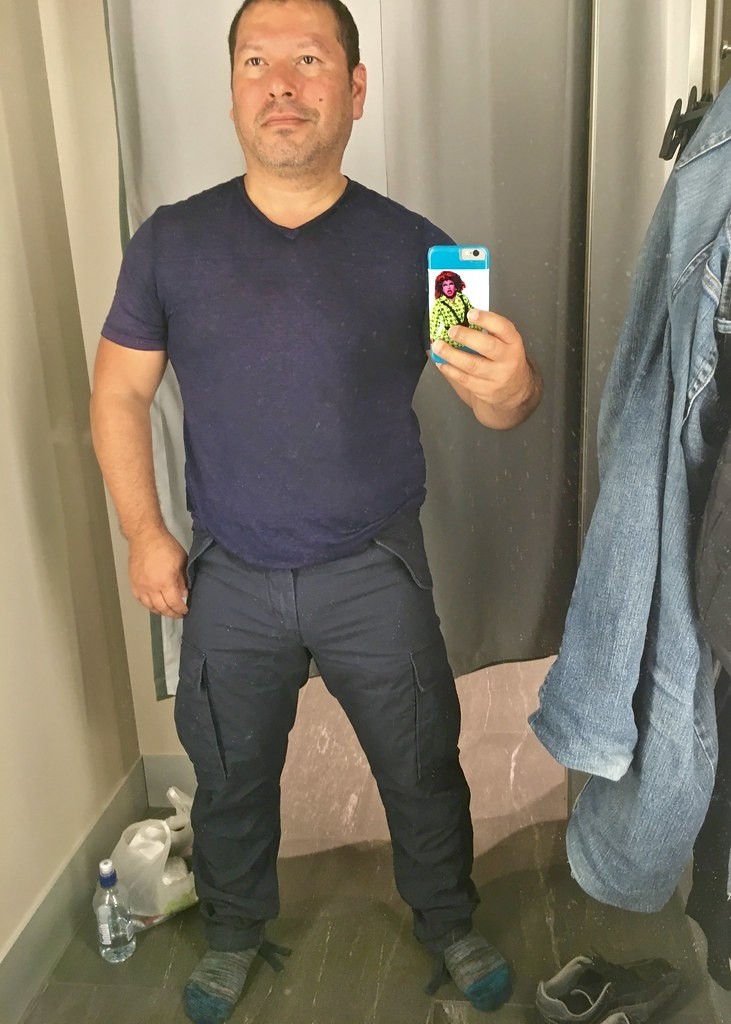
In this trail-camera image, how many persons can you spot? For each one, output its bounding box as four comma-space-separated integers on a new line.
88, 1, 544, 1023
429, 271, 482, 348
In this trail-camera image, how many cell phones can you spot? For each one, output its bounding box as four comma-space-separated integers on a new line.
428, 244, 490, 362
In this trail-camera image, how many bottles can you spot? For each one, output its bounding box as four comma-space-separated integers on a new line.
93, 859, 135, 964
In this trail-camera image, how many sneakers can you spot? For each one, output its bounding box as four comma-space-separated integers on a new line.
536, 943, 679, 1024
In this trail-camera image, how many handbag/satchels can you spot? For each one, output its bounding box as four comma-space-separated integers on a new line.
108, 786, 201, 934
